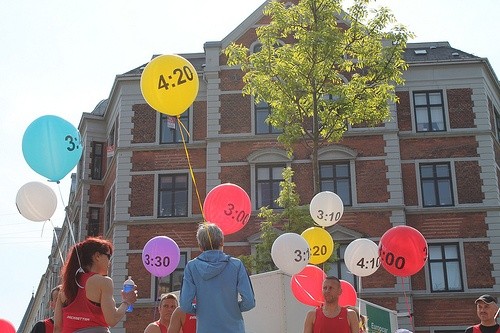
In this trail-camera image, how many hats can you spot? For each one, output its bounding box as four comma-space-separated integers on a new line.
475, 294, 497, 305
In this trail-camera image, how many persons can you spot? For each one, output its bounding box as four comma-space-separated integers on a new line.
303, 276, 367, 333
180, 223, 256, 333
465, 294, 500, 333
144, 294, 196, 333
53, 238, 138, 333
31, 285, 61, 333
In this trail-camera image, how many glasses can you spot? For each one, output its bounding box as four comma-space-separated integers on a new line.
99, 251, 111, 260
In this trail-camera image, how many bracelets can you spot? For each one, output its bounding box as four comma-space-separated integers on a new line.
121, 301, 128, 305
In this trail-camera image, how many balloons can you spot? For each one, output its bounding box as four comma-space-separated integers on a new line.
16, 182, 57, 221
271, 232, 310, 274
21, 115, 82, 184
140, 53, 199, 116
344, 238, 380, 276
301, 227, 334, 265
378, 225, 428, 276
142, 236, 180, 278
204, 184, 251, 236
310, 191, 344, 226
0, 318, 17, 333
338, 280, 357, 308
290, 265, 327, 307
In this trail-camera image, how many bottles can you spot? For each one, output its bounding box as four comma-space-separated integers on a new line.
122, 276, 135, 312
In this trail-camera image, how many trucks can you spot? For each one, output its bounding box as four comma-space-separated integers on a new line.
160, 269, 398, 333
